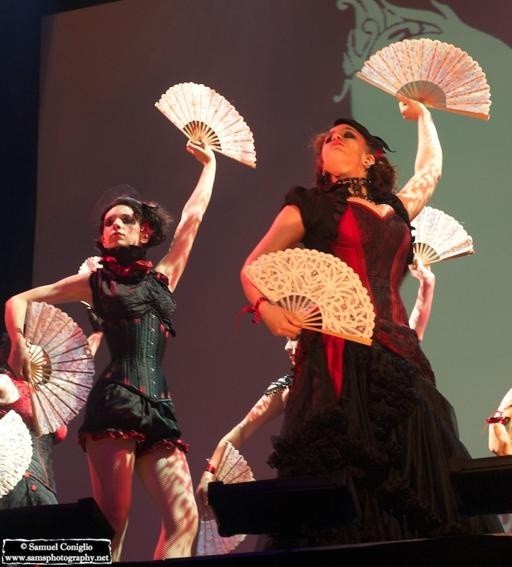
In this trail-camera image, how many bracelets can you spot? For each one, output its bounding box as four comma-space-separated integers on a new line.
247, 294, 273, 325
5, 328, 27, 339
483, 413, 510, 430
201, 459, 219, 478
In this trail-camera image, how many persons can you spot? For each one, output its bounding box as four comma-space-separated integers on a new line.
237, 90, 475, 564
2, 135, 218, 566
192, 258, 437, 549
1, 329, 103, 508
482, 374, 512, 459
